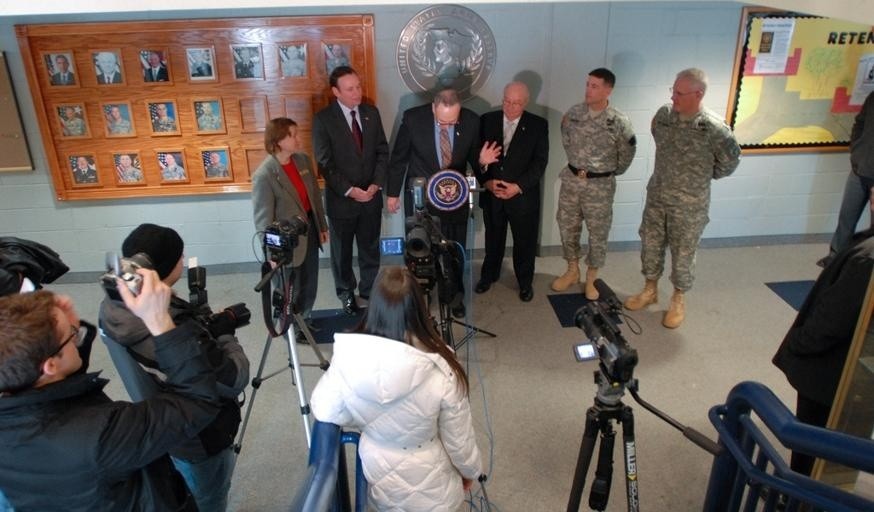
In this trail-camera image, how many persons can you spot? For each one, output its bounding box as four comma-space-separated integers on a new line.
625, 68, 741, 328
0, 268, 222, 512
234, 48, 254, 78
250, 118, 330, 340
98, 223, 249, 512
327, 44, 347, 75
551, 67, 636, 301
759, 186, 874, 507
310, 266, 482, 512
0, 237, 69, 297
816, 91, 874, 269
312, 66, 389, 318
282, 46, 306, 76
387, 90, 502, 318
36, 41, 231, 184
474, 82, 549, 303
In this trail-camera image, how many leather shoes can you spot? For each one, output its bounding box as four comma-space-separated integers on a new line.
343, 295, 358, 316
475, 279, 496, 294
519, 285, 533, 302
452, 301, 465, 318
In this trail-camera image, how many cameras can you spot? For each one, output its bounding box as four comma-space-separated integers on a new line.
98, 252, 152, 304
188, 257, 251, 326
254, 215, 308, 293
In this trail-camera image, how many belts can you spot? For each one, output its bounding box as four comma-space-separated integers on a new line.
568, 163, 614, 178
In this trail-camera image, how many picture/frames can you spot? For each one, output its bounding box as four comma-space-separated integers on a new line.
145, 99, 182, 137
321, 39, 355, 79
230, 44, 265, 82
110, 150, 147, 187
152, 149, 190, 184
64, 152, 103, 188
89, 48, 127, 88
183, 44, 219, 84
199, 147, 233, 182
1, 52, 35, 172
725, 7, 874, 153
13, 13, 378, 203
53, 103, 93, 141
276, 41, 310, 80
98, 101, 137, 139
190, 96, 227, 135
136, 46, 174, 86
39, 49, 80, 89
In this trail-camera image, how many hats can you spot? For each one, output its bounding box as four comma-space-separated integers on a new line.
122, 224, 183, 281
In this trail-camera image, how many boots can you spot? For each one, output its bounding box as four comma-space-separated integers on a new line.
624, 279, 657, 310
551, 258, 581, 292
662, 288, 687, 329
585, 267, 600, 300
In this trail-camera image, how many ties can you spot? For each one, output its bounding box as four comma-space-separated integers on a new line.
440, 131, 453, 170
107, 76, 110, 84
504, 121, 515, 158
153, 70, 156, 81
62, 75, 65, 82
350, 110, 362, 154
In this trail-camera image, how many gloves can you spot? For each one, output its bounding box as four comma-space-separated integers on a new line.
210, 313, 235, 339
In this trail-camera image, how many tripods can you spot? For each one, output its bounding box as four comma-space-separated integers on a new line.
421, 281, 496, 354
566, 399, 641, 511
234, 267, 328, 478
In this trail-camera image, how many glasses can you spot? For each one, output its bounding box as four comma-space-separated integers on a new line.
436, 114, 460, 126
502, 98, 528, 109
669, 87, 698, 98
49, 326, 78, 360
